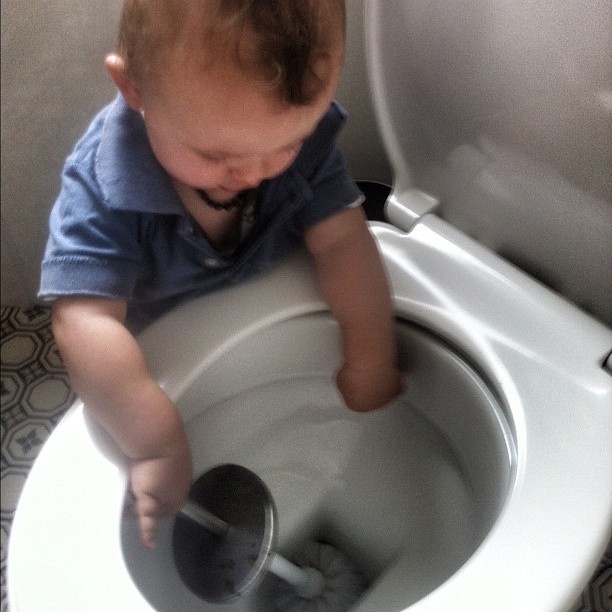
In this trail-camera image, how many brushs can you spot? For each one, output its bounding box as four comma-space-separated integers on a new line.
173, 462, 364, 607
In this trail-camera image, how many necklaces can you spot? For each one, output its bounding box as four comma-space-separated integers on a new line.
191, 185, 252, 212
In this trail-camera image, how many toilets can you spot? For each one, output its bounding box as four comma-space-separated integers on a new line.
1, 1, 610, 611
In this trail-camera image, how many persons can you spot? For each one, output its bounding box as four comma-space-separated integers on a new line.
38, 0, 409, 548
